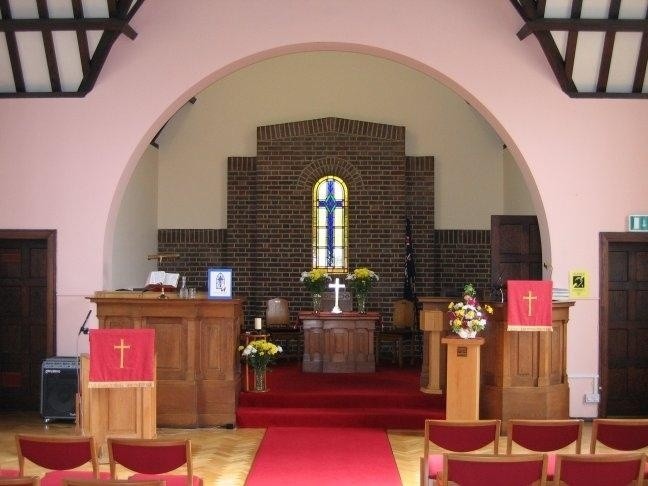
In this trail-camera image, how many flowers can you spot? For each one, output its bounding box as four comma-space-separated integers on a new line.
448, 282, 493, 331
297, 268, 330, 299
237, 337, 283, 375
347, 267, 378, 301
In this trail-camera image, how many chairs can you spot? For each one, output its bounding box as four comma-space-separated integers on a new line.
415, 417, 501, 486
60, 477, 168, 486
505, 414, 582, 480
16, 432, 115, 484
106, 433, 207, 486
392, 298, 423, 365
1, 476, 41, 486
440, 451, 549, 485
586, 416, 647, 460
263, 298, 300, 368
552, 449, 647, 486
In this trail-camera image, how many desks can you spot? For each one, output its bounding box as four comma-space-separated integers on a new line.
375, 331, 405, 368
243, 330, 275, 392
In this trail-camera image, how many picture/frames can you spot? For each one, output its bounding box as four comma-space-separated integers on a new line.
207, 268, 233, 300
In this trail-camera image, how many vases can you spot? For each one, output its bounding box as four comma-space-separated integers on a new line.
458, 328, 477, 340
356, 294, 368, 316
253, 365, 266, 392
312, 291, 322, 316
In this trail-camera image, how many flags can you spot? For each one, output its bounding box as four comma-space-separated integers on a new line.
88, 328, 155, 388
506, 280, 553, 332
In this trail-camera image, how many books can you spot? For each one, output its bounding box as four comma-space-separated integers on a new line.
552, 289, 569, 300
144, 271, 180, 289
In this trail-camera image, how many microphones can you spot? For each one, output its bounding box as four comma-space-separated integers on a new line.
78, 309, 92, 333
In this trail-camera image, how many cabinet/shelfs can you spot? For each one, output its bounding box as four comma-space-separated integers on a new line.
294, 310, 378, 376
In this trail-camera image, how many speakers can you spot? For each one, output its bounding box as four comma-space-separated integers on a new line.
40, 357, 77, 424
